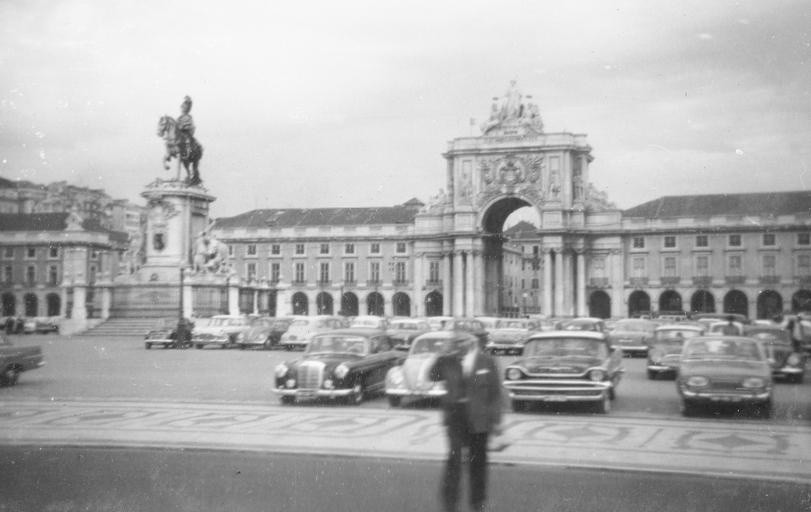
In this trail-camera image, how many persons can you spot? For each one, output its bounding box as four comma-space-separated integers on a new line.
721, 315, 740, 336
5, 316, 14, 335
425, 319, 505, 512
15, 314, 24, 335
170, 94, 195, 158
784, 315, 804, 351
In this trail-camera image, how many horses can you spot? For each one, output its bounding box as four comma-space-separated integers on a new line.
156, 114, 203, 185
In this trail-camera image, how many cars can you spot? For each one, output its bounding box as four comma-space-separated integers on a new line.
676, 337, 771, 414
0, 328, 46, 387
270, 328, 408, 405
743, 324, 804, 382
503, 330, 622, 415
23, 319, 58, 334
384, 330, 466, 408
642, 325, 706, 380
